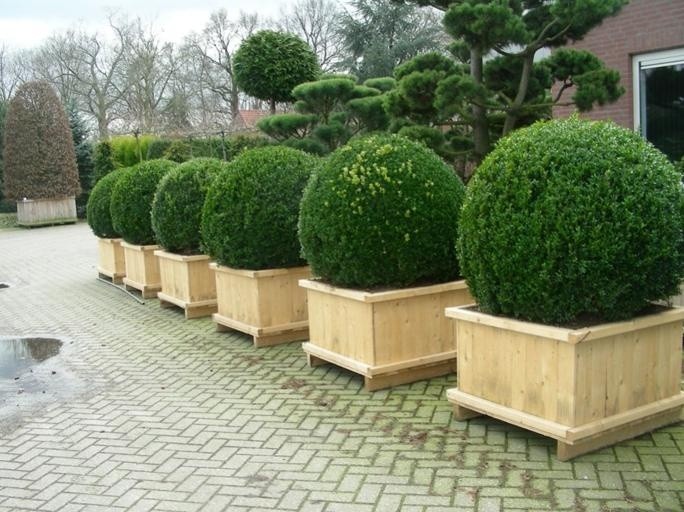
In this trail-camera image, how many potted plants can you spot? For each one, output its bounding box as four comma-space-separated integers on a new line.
1, 77, 82, 230
